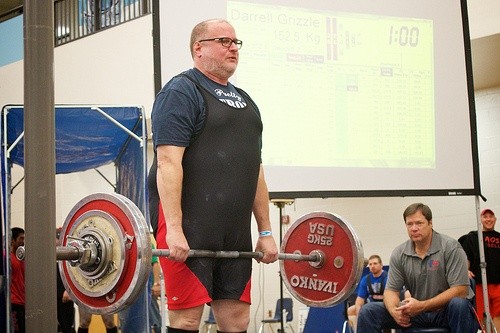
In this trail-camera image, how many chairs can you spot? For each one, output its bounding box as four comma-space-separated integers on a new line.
404, 325, 448, 333
204, 307, 219, 333
261, 298, 295, 333
341, 294, 356, 333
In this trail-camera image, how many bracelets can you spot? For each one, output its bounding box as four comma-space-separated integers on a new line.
258, 230, 271, 237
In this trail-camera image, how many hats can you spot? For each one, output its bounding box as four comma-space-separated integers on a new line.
480, 207, 496, 217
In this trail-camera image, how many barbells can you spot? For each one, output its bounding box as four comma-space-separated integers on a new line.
16, 193, 365, 316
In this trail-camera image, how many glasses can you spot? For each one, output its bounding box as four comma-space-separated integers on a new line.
198, 36, 242, 50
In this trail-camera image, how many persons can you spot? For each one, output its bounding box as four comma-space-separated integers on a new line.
347, 255, 387, 333
3, 227, 118, 333
357, 203, 472, 333
458, 208, 500, 333
147, 241, 162, 333
148, 19, 278, 333
